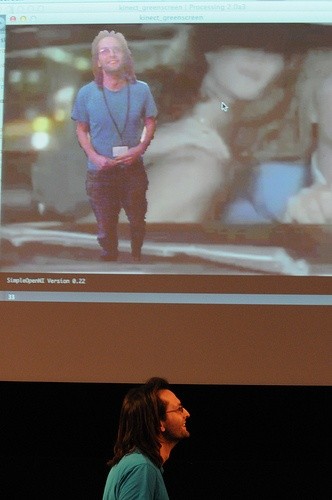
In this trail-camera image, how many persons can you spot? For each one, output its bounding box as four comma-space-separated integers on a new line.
101, 376, 191, 500
71, 29, 159, 261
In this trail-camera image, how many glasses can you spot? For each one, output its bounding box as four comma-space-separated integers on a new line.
165, 404, 184, 415
98, 48, 124, 56
